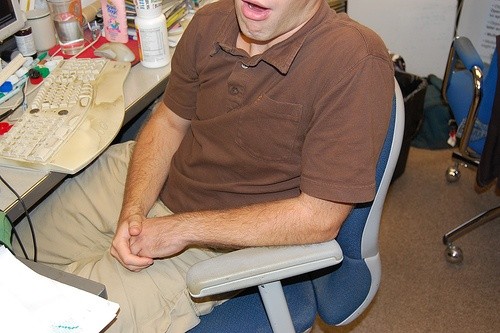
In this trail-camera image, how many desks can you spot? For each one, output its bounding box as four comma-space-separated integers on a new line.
0, 46, 176, 300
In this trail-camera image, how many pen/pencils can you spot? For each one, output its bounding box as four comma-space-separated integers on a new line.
102, 32, 137, 41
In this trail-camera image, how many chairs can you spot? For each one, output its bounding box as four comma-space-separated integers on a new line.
443, 35, 500, 263
120, 74, 405, 333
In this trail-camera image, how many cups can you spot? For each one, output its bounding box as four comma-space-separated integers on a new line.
25, 11, 56, 51
47, 0, 85, 55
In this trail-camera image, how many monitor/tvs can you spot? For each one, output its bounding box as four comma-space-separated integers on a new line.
0, 0, 25, 87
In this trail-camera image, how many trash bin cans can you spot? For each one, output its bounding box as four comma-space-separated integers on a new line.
390, 68, 427, 184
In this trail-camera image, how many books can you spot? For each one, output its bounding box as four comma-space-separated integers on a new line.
96, 0, 197, 49
0, 244, 120, 333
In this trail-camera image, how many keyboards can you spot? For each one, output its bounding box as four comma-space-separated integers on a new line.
0, 58, 132, 175
93, 42, 136, 62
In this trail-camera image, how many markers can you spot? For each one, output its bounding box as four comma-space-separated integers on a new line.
23, 51, 47, 77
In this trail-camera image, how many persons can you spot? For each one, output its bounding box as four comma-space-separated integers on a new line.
11, 0, 394, 333
